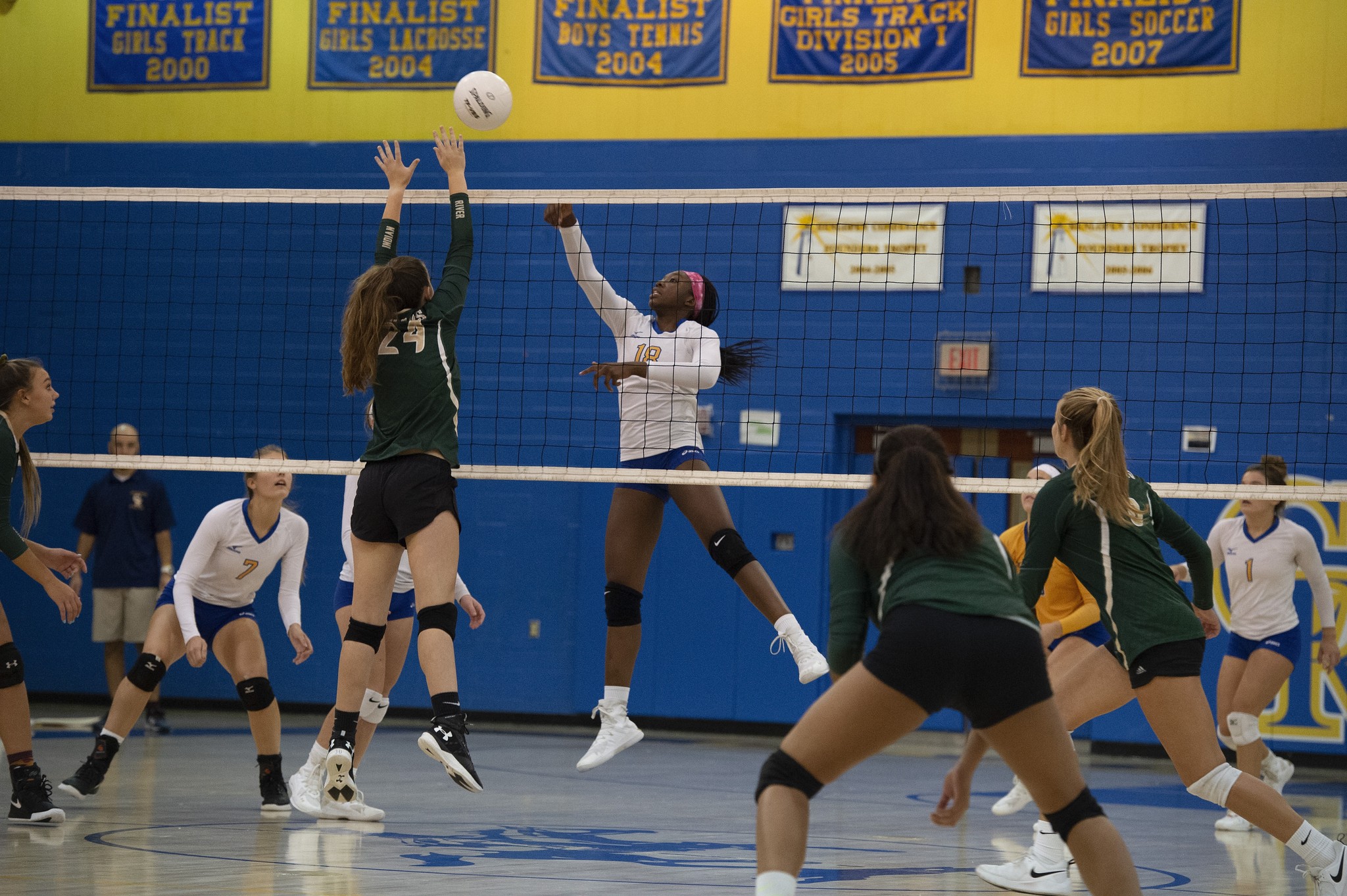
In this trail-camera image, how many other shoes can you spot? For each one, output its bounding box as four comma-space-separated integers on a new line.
92, 710, 110, 730
142, 706, 172, 733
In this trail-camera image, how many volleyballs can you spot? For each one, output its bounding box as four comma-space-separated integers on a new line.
453, 70, 512, 131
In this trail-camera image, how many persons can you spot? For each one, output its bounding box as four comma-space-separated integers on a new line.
327, 127, 484, 806
0, 358, 87, 824
998, 461, 1114, 817
1168, 454, 1341, 833
71, 422, 174, 737
285, 397, 489, 822
974, 386, 1347, 895
754, 425, 1145, 896
543, 204, 832, 771
56, 445, 313, 811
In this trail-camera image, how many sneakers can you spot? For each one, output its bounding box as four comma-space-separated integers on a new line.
8, 774, 66, 823
1214, 809, 1259, 831
256, 764, 292, 811
319, 789, 386, 821
57, 756, 106, 799
976, 851, 1072, 896
575, 696, 644, 773
1295, 832, 1347, 896
287, 762, 325, 817
1263, 757, 1295, 795
322, 729, 358, 803
769, 623, 829, 684
990, 773, 1033, 816
418, 709, 483, 792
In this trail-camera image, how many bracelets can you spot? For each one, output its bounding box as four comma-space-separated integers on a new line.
160, 565, 174, 576
559, 213, 577, 227
